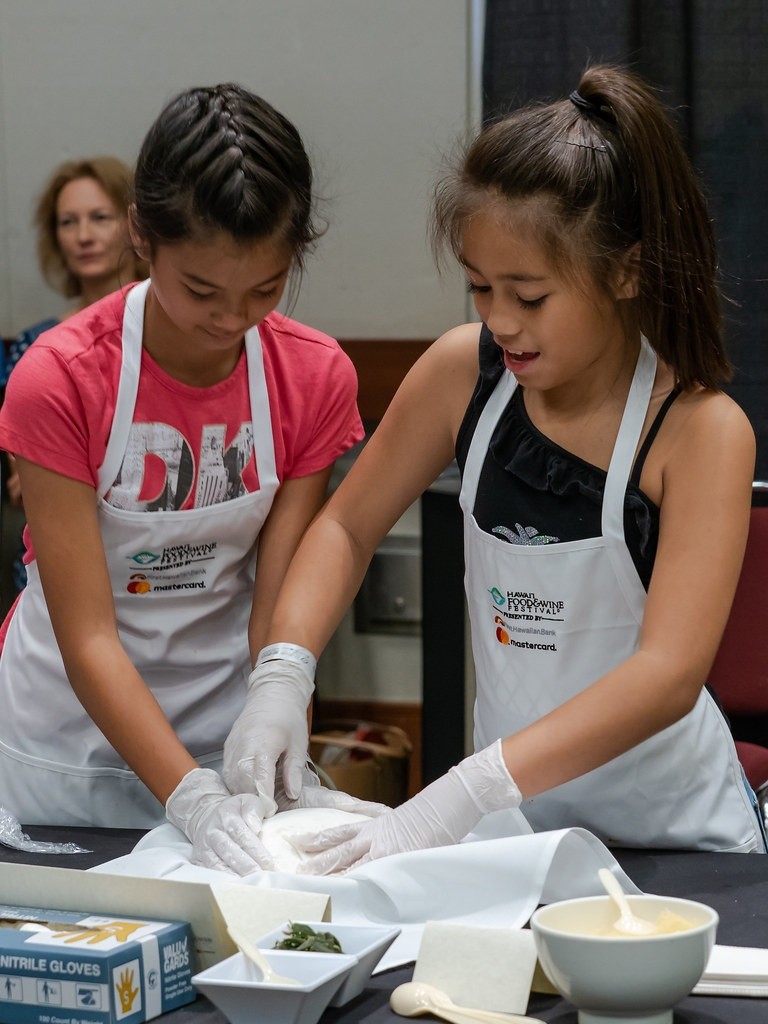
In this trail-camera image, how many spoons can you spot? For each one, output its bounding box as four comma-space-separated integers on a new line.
598, 868, 657, 936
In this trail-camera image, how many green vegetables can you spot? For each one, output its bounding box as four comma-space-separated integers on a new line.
267, 922, 342, 954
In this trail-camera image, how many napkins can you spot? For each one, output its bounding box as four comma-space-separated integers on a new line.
690, 944, 768, 998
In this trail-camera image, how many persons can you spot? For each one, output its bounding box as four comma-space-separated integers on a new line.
0, 85, 367, 878
1, 155, 146, 590
223, 64, 768, 877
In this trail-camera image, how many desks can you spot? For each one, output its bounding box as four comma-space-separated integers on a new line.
0, 819, 768, 1024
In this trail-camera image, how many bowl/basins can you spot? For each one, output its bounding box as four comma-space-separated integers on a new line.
252, 920, 402, 1008
529, 894, 719, 1024
190, 948, 358, 1024
